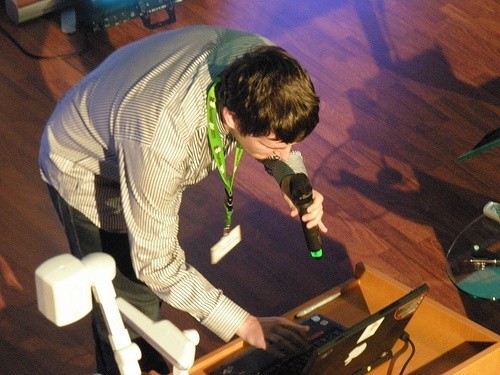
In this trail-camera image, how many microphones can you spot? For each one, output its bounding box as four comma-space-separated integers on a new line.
290, 172, 323, 257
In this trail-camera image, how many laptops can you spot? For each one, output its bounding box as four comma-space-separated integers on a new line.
208, 283, 429, 375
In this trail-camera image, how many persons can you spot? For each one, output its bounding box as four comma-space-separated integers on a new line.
39, 25, 328, 375
